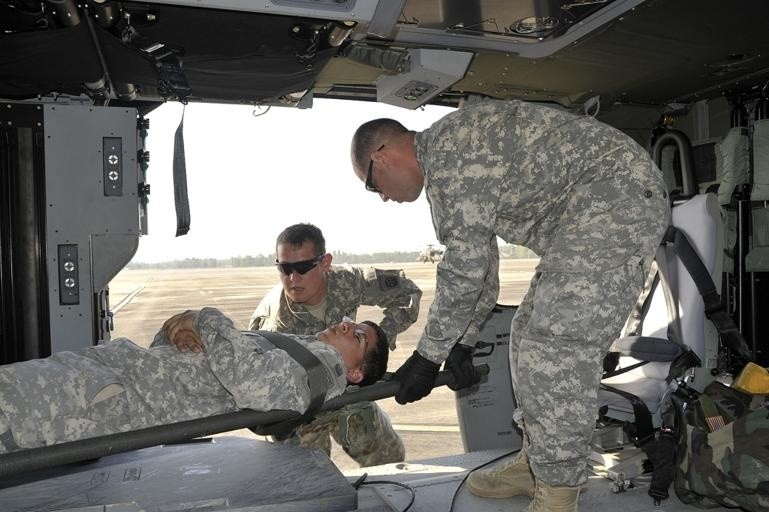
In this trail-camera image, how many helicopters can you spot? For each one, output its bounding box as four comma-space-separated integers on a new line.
417, 244, 442, 263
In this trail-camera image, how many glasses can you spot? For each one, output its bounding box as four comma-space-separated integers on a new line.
275, 254, 325, 275
365, 144, 385, 193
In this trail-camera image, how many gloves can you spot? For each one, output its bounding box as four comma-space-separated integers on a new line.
388, 350, 441, 405
444, 343, 482, 392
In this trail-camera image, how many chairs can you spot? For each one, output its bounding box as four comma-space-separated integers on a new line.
597, 190, 729, 493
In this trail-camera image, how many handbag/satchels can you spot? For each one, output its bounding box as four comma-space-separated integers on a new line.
648, 380, 768, 512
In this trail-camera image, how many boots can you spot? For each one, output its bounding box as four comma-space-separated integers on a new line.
466, 422, 536, 499
523, 479, 580, 512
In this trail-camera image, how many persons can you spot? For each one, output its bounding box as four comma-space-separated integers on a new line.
2, 305, 390, 456
349, 98, 672, 512
246, 221, 422, 470
